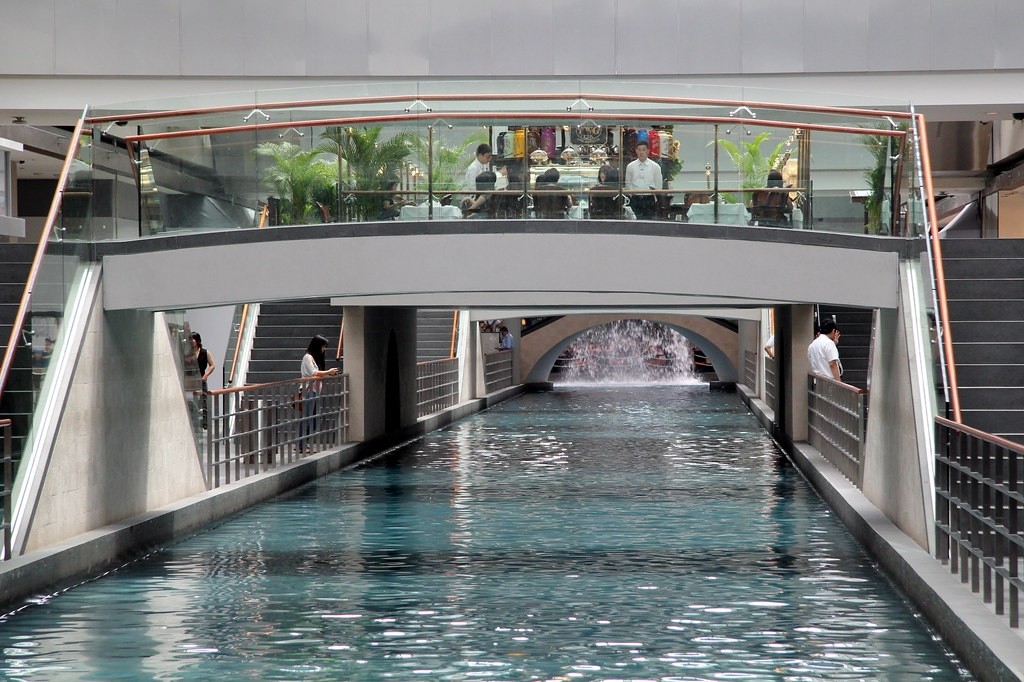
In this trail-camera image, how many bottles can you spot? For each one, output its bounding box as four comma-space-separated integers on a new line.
624, 128, 669, 159
496, 129, 555, 159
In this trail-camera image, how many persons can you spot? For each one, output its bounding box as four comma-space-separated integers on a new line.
297, 335, 338, 456
190, 332, 215, 431
32, 337, 53, 392
765, 318, 842, 382
481, 320, 514, 351
379, 140, 792, 227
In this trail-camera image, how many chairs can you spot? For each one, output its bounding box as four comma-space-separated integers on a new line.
751, 204, 793, 227
589, 186, 711, 224
466, 189, 526, 219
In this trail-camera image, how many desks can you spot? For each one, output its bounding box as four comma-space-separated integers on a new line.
787, 209, 804, 228
686, 203, 749, 226
400, 205, 461, 221
530, 206, 586, 220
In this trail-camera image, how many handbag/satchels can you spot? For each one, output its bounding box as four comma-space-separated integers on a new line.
292, 391, 303, 410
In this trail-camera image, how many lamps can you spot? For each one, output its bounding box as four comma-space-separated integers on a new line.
11, 116, 27, 124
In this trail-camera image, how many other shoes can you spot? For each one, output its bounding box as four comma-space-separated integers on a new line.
299, 447, 316, 453
